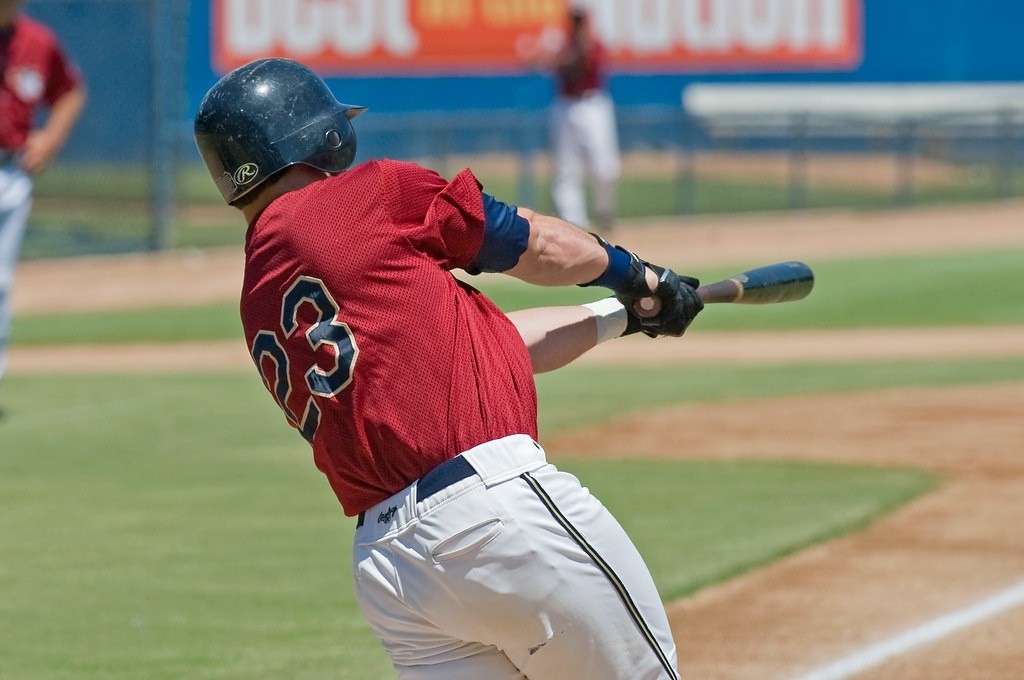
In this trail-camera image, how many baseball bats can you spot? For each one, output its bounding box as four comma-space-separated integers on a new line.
637, 262, 814, 317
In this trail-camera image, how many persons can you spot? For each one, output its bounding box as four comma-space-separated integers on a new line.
1, 0, 83, 374
192, 56, 705, 680
548, 11, 619, 233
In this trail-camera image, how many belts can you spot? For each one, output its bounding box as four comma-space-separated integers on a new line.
357, 455, 477, 530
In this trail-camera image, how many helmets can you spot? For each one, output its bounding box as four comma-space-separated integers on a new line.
193, 56, 369, 206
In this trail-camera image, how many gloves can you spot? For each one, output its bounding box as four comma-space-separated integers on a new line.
584, 275, 705, 346
575, 231, 683, 327
